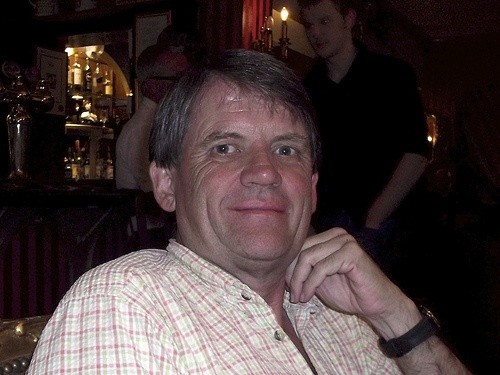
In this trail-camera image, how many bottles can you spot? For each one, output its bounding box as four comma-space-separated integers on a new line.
68, 139, 114, 180
82, 57, 92, 91
72, 54, 82, 91
103, 71, 112, 96
92, 63, 103, 92
68, 59, 73, 92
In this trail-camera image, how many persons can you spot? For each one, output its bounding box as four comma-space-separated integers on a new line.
301, 0, 431, 308
26, 50, 469, 375
115, 24, 208, 256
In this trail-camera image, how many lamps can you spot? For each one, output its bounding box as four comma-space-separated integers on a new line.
266, 6, 291, 57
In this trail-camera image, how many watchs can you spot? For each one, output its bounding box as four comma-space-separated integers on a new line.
378, 306, 444, 358
